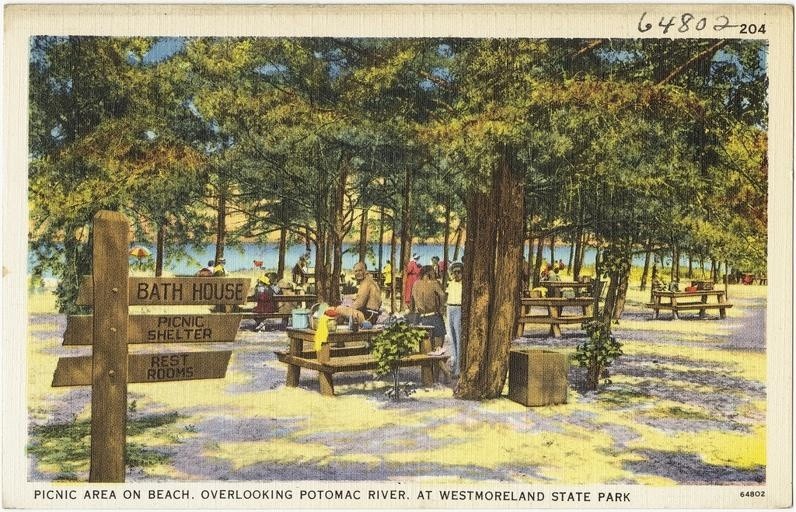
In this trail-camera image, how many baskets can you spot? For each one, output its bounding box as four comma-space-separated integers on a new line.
309, 315, 337, 331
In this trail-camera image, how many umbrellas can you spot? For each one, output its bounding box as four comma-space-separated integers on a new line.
129, 245, 151, 267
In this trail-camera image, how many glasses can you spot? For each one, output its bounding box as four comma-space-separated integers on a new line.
453, 270, 462, 273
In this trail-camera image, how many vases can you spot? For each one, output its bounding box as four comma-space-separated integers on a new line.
743, 272, 754, 285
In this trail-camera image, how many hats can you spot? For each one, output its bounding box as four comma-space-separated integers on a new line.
259, 275, 270, 285
449, 261, 464, 270
218, 258, 226, 262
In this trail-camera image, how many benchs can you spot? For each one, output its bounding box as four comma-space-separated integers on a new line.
242, 269, 733, 391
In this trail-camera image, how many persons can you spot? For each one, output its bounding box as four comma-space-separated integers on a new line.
522, 257, 564, 282
384, 253, 464, 379
253, 249, 311, 331
334, 261, 382, 325
213, 257, 229, 276
206, 260, 215, 274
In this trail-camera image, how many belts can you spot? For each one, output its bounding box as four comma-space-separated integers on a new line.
447, 304, 462, 306
